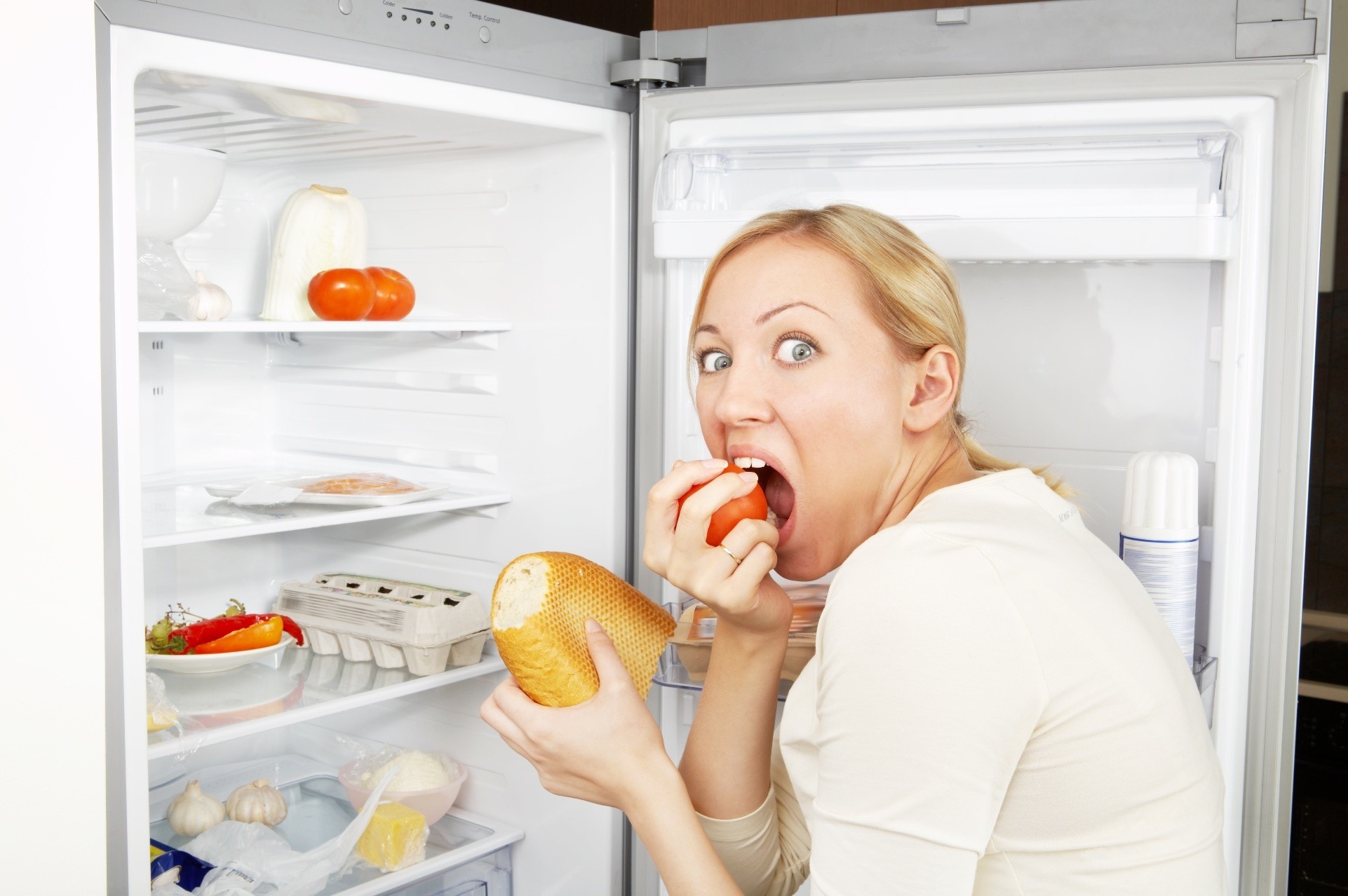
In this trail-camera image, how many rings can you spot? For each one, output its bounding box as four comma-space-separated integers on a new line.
717, 541, 743, 566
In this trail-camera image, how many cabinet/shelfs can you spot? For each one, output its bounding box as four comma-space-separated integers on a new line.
129, 65, 556, 896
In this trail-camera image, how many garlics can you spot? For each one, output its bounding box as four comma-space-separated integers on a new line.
225, 778, 288, 827
165, 777, 225, 836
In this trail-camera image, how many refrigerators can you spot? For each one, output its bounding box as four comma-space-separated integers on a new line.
0, 1, 1341, 893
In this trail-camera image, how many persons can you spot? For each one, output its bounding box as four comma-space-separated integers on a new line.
478, 192, 1227, 895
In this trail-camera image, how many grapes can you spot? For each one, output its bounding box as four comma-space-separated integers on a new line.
145, 598, 246, 655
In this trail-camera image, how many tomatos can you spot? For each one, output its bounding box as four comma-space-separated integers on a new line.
364, 266, 416, 322
306, 267, 376, 322
677, 461, 767, 546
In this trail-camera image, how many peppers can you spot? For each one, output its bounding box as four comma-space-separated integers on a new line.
151, 613, 304, 654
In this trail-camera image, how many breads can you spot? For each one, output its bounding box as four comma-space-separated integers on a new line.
490, 551, 678, 708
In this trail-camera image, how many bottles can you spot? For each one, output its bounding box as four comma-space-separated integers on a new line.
1118, 451, 1199, 674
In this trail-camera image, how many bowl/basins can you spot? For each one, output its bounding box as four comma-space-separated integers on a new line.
339, 754, 469, 829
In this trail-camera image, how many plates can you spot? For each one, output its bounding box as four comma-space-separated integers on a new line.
145, 632, 295, 676
204, 476, 444, 507
